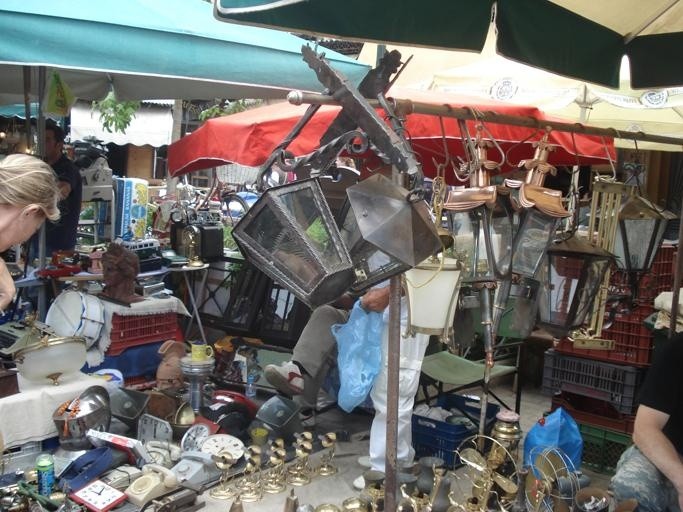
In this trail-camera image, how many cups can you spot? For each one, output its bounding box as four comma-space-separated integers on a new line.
191, 340, 214, 361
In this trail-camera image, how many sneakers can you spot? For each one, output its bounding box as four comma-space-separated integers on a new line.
264, 360, 305, 395
263, 411, 316, 431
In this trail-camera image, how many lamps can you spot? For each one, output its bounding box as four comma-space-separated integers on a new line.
12, 332, 88, 387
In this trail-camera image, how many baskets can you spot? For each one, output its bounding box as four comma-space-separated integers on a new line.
412, 394, 501, 470
83, 344, 165, 385
551, 391, 635, 435
609, 242, 675, 305
105, 313, 185, 357
553, 303, 654, 368
543, 412, 634, 476
542, 348, 646, 415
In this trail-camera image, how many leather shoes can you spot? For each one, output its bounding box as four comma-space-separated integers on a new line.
353, 467, 385, 491
358, 456, 414, 474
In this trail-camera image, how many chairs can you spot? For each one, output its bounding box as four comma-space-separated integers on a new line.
413, 295, 535, 457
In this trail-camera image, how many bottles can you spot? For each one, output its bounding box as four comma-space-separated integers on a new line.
244, 374, 257, 404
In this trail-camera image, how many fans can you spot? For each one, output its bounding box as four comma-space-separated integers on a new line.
236, 430, 337, 503
452, 434, 580, 510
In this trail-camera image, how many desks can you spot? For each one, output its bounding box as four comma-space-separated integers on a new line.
51, 263, 216, 343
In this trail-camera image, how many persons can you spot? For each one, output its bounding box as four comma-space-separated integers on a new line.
29, 119, 82, 258
0, 153, 59, 314
262, 247, 405, 428
609, 337, 683, 507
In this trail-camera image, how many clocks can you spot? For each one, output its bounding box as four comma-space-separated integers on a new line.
66, 414, 247, 509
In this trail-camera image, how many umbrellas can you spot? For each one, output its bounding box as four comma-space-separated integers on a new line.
359, 42, 682, 152
213, 0, 681, 91
167, 96, 616, 188
